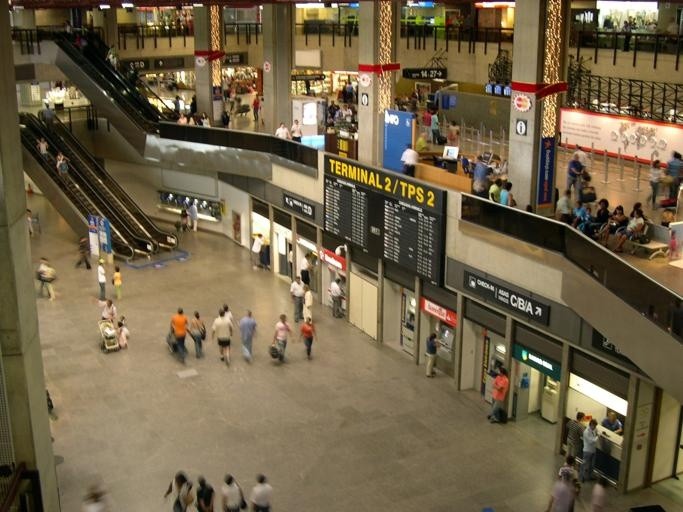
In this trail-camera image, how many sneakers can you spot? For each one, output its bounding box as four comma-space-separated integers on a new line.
426, 372, 436, 378
294, 316, 304, 322
251, 264, 262, 272
220, 356, 230, 368
332, 314, 343, 318
486, 415, 498, 425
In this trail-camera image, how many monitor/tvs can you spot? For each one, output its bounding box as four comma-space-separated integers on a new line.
443, 146, 459, 159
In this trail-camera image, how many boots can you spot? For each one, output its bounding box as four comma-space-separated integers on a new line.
611, 234, 626, 252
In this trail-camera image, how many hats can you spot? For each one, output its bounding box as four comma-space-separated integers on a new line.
99, 258, 106, 264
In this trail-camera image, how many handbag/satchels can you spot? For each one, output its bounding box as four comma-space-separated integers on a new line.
234, 480, 248, 510
581, 169, 591, 181
495, 407, 508, 423
268, 342, 279, 359
35, 270, 56, 282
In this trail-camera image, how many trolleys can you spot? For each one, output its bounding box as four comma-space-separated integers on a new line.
573, 173, 602, 214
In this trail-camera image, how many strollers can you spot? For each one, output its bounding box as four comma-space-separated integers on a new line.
98, 318, 120, 353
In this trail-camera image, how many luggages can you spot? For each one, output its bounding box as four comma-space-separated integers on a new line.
578, 186, 596, 202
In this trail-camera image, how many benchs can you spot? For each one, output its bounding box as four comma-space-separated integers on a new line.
601, 217, 671, 260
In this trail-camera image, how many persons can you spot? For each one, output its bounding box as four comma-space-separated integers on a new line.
425, 333, 441, 378
196, 476, 215, 512
544, 466, 577, 512
564, 412, 586, 466
487, 367, 509, 424
221, 473, 244, 512
601, 412, 623, 435
250, 473, 273, 512
164, 471, 192, 512
578, 419, 602, 483
27, 13, 683, 366
564, 455, 578, 479
